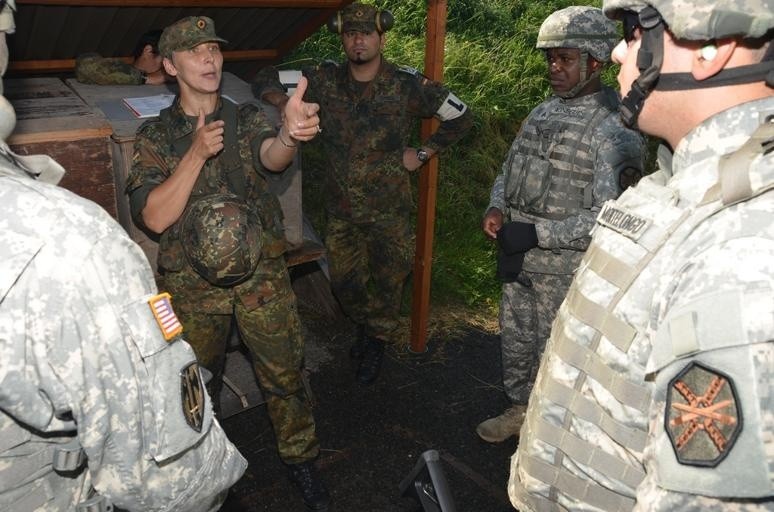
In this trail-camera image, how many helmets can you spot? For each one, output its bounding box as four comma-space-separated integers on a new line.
535, 6, 620, 65
177, 195, 264, 288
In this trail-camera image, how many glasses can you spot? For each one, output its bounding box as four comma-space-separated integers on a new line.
622, 10, 640, 42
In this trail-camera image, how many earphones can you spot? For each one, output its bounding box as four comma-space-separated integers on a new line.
701, 45, 717, 62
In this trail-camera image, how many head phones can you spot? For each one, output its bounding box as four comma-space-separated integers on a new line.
326, 9, 395, 32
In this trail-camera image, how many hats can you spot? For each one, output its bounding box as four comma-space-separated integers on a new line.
341, 3, 376, 34
158, 16, 229, 60
601, 0, 774, 42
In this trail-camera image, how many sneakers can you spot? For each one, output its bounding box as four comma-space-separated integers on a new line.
349, 323, 390, 387
475, 404, 528, 443
287, 462, 334, 511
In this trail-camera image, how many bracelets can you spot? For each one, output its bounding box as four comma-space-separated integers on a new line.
138, 70, 146, 82
273, 125, 299, 152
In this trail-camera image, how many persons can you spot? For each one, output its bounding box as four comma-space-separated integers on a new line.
505, 2, 774, 511
0, 0, 251, 511
475, 4, 648, 444
253, 3, 469, 387
75, 26, 178, 84
125, 15, 336, 511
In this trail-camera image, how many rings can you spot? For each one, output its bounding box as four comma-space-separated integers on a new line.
316, 126, 321, 133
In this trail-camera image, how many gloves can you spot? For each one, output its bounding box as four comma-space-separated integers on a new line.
495, 221, 538, 282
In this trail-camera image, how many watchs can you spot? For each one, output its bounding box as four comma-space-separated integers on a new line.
412, 146, 431, 164
